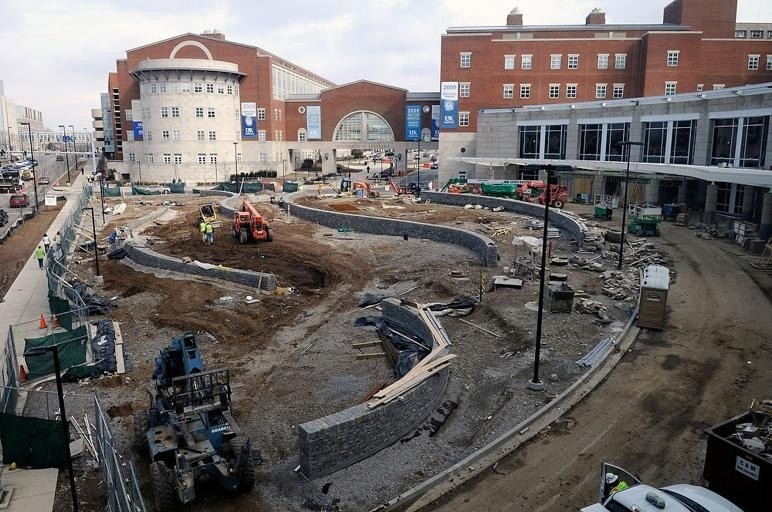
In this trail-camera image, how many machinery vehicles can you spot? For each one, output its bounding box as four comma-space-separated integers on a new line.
316, 176, 421, 198
230, 200, 273, 245
520, 181, 569, 207
130, 329, 258, 512
627, 203, 663, 237
196, 203, 224, 229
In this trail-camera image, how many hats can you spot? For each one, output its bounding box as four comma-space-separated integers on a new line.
606, 473, 618, 484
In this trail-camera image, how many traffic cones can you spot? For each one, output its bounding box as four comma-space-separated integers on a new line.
17, 363, 29, 383
50, 312, 60, 328
38, 313, 49, 329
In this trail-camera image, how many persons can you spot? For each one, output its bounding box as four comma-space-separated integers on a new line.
43, 234, 49, 253
606, 473, 628, 496
53, 231, 60, 245
199, 220, 214, 246
34, 245, 45, 270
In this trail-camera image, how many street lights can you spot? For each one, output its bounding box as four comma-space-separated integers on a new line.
78, 206, 103, 287
20, 121, 40, 211
616, 141, 647, 270
307, 154, 312, 177
232, 141, 240, 194
525, 160, 579, 392
137, 160, 142, 187
283, 159, 287, 185
7, 126, 13, 153
413, 138, 423, 199
295, 155, 299, 180
58, 124, 71, 183
69, 124, 79, 170
83, 127, 90, 158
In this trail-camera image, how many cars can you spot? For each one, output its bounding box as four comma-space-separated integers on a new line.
372, 150, 438, 170
144, 184, 171, 194
0, 148, 65, 227
570, 462, 747, 512
